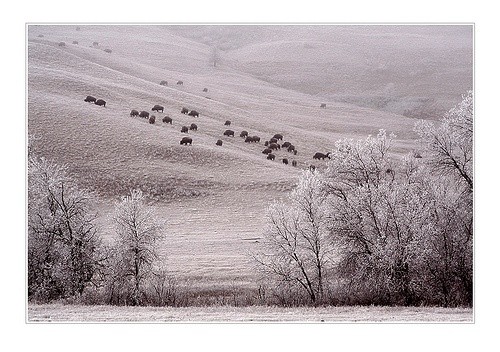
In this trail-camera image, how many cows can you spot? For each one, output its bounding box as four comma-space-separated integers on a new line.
148, 115, 156, 124
95, 98, 107, 107
224, 130, 234, 137
152, 104, 165, 113
245, 136, 254, 144
181, 125, 188, 134
240, 131, 248, 138
283, 158, 288, 165
267, 153, 276, 162
320, 103, 326, 108
162, 115, 172, 124
292, 159, 297, 168
188, 122, 198, 131
179, 137, 192, 146
139, 110, 149, 119
313, 152, 331, 161
224, 120, 231, 126
216, 140, 223, 146
188, 110, 200, 118
157, 78, 208, 94
84, 96, 96, 104
130, 109, 139, 117
253, 135, 261, 143
262, 134, 297, 155
181, 107, 188, 115
37, 26, 112, 53
